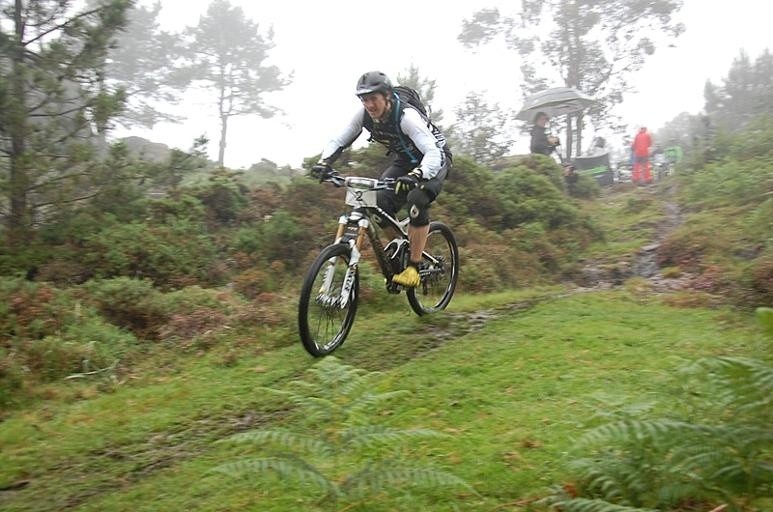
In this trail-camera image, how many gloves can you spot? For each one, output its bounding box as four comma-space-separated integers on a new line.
394, 174, 417, 195
311, 163, 329, 184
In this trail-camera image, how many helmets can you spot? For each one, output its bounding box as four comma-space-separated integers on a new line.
356, 71, 392, 98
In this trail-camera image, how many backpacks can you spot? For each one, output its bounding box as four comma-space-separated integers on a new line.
390, 86, 427, 134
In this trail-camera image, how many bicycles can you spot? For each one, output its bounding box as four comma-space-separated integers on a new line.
296, 166, 460, 360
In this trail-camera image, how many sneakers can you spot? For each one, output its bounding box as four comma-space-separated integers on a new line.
392, 268, 420, 287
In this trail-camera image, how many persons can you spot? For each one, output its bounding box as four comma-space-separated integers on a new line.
529, 111, 558, 156
630, 127, 652, 185
663, 138, 682, 177
310, 70, 453, 286
652, 141, 666, 181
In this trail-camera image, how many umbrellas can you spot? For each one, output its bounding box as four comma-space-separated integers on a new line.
514, 86, 595, 124
